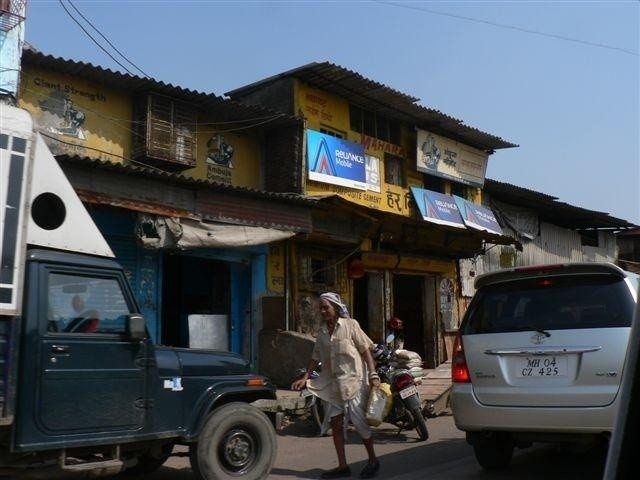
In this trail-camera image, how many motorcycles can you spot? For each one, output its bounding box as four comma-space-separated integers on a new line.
297, 334, 430, 441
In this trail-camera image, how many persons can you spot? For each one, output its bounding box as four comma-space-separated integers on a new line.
291, 292, 380, 479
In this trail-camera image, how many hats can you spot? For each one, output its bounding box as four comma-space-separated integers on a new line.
318, 291, 350, 319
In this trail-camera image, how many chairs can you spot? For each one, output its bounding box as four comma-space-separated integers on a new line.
524, 296, 560, 323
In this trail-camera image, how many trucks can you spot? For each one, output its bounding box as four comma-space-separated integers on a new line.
1, 104, 279, 480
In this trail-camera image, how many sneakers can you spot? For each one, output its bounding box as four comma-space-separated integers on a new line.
322, 467, 353, 480
359, 461, 380, 480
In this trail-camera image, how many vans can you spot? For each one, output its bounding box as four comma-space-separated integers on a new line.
450, 261, 640, 470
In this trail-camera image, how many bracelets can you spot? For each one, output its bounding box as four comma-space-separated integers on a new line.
368, 371, 380, 380
